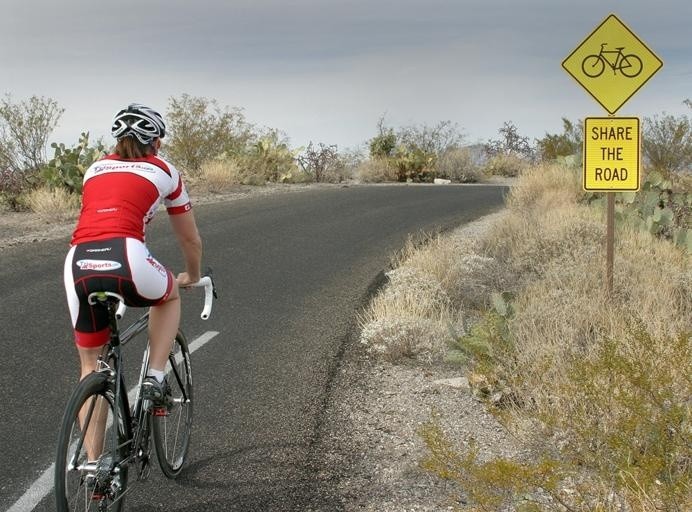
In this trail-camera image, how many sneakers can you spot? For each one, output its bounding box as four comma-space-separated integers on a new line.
81, 461, 111, 501
143, 374, 176, 409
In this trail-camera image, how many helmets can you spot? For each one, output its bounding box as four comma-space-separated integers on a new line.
111, 102, 166, 143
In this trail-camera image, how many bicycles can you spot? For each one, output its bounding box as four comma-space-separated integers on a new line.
55, 276, 213, 512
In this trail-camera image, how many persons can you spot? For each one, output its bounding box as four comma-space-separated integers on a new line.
61, 103, 204, 501
